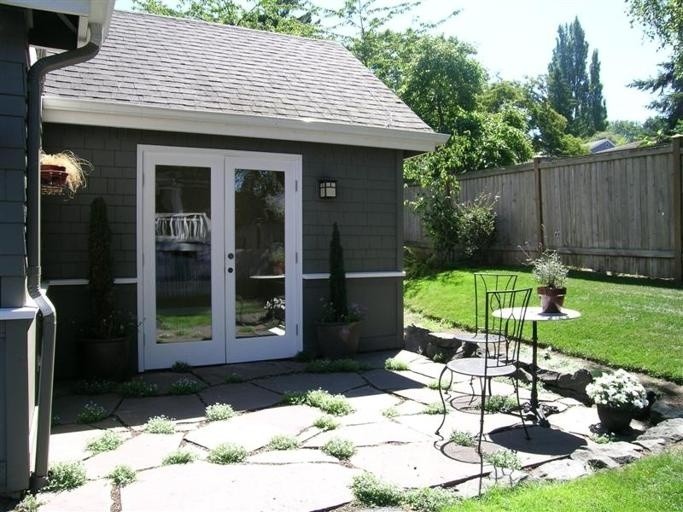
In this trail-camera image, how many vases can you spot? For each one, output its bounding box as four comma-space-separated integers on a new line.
596, 404, 635, 434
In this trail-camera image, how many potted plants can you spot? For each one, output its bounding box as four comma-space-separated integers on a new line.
311, 221, 363, 359
40, 149, 95, 197
60, 197, 132, 383
532, 247, 569, 315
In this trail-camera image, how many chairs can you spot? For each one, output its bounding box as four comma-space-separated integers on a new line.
435, 272, 533, 454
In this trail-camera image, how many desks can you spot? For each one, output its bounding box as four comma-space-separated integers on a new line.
490, 306, 583, 428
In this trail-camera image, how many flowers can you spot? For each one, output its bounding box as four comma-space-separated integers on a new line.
585, 367, 650, 410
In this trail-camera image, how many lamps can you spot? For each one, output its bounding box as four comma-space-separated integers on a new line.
318, 176, 337, 199
